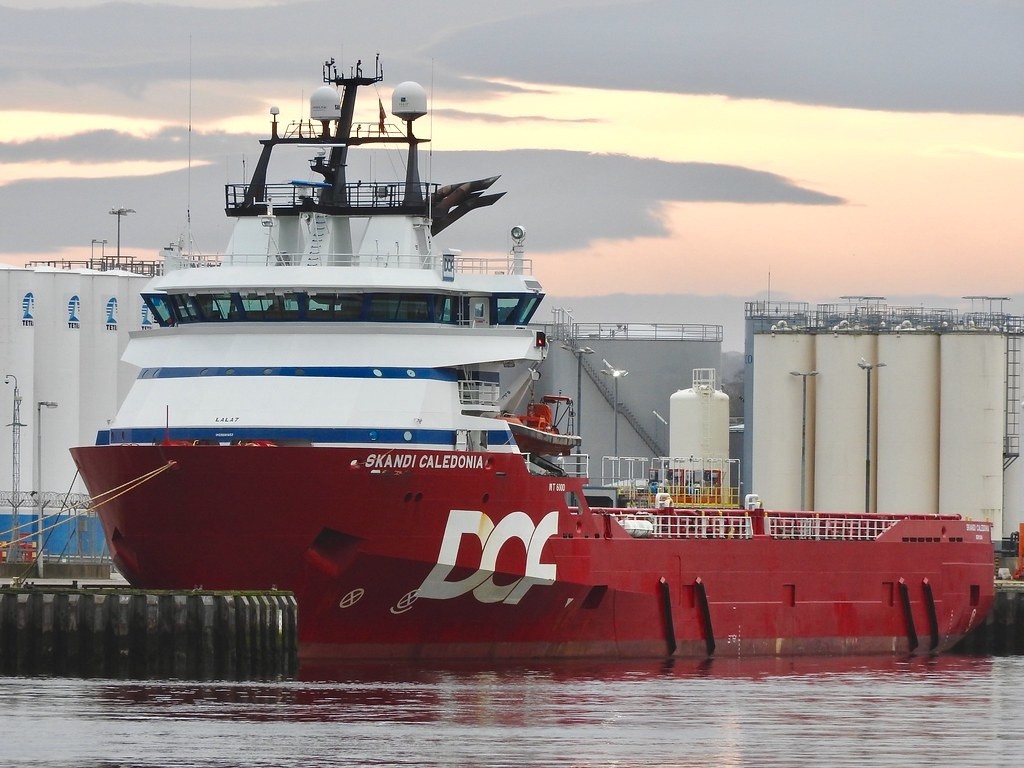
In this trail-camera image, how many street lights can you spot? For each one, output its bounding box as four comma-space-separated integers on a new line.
37, 401, 58, 579
789, 371, 818, 509
858, 361, 887, 514
108, 206, 137, 268
561, 345, 595, 476
600, 359, 629, 457
5, 375, 27, 562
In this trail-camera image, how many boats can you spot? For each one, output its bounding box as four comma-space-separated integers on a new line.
69, 31, 994, 661
